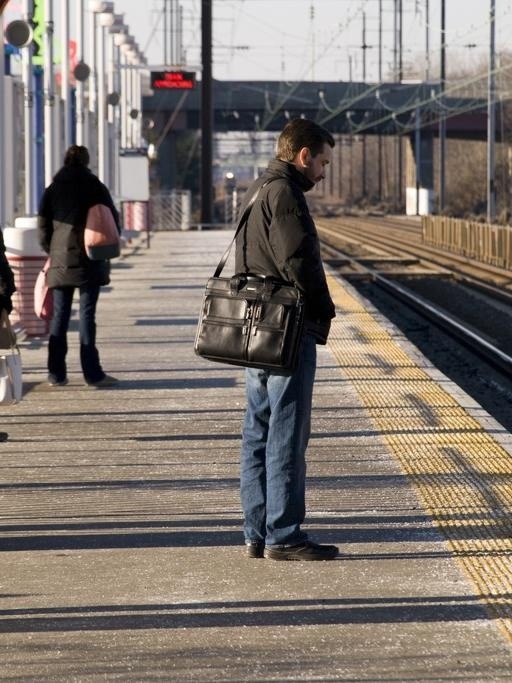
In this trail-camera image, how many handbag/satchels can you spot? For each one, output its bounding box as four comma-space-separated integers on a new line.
193, 272, 304, 376
84, 205, 119, 260
0, 354, 23, 407
33, 270, 52, 322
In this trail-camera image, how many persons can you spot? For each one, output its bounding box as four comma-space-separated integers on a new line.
0, 225, 18, 444
233, 119, 341, 564
36, 145, 123, 389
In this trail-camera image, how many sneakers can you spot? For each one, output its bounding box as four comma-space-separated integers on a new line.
246, 539, 338, 562
48, 377, 69, 386
86, 375, 119, 386
